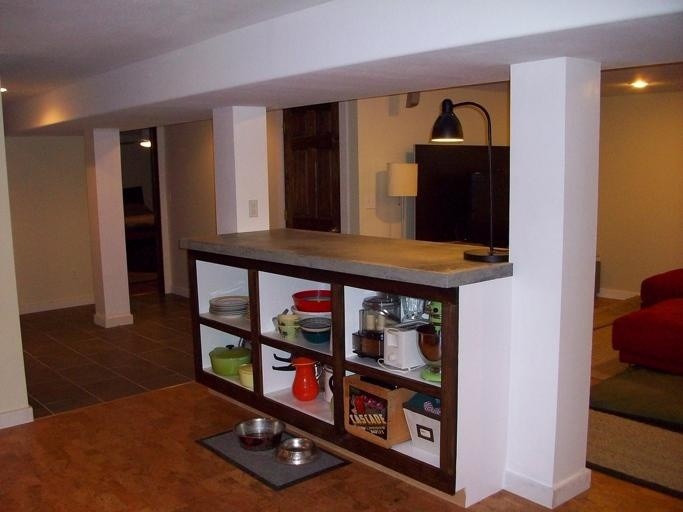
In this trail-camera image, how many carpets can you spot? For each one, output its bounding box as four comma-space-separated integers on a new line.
195, 425, 353, 493
586, 293, 683, 500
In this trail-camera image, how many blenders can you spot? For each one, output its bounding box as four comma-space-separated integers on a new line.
414, 301, 443, 384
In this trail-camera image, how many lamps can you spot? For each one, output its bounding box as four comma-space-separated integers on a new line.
383, 102, 510, 263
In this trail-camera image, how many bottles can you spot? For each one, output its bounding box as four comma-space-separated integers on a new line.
323, 364, 334, 403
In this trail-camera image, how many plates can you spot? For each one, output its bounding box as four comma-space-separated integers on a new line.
208, 295, 250, 319
298, 316, 332, 332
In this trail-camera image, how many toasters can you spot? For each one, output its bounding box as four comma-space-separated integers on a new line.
382, 319, 428, 370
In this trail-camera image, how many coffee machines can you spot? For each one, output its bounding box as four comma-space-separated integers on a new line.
351, 294, 401, 364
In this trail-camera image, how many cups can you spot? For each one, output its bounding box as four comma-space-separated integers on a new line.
277, 314, 300, 339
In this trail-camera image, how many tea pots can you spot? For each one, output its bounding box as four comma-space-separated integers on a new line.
289, 354, 323, 402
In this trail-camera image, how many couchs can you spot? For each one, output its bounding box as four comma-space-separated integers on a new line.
613, 259, 682, 366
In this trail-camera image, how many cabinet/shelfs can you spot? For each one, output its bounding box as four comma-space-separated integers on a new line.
189, 222, 513, 499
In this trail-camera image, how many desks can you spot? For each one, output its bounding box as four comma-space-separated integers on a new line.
0, 381, 474, 512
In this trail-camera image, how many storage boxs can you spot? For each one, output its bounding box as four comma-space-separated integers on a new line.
343, 371, 413, 450
403, 392, 443, 455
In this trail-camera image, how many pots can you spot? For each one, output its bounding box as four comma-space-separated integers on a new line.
237, 363, 254, 388
208, 343, 251, 377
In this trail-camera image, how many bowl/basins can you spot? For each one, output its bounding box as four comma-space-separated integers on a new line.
291, 289, 332, 312
290, 305, 331, 318
301, 331, 330, 344
232, 416, 286, 451
272, 437, 321, 465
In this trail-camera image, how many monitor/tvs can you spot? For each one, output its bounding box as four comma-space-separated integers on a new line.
414, 142, 509, 248
